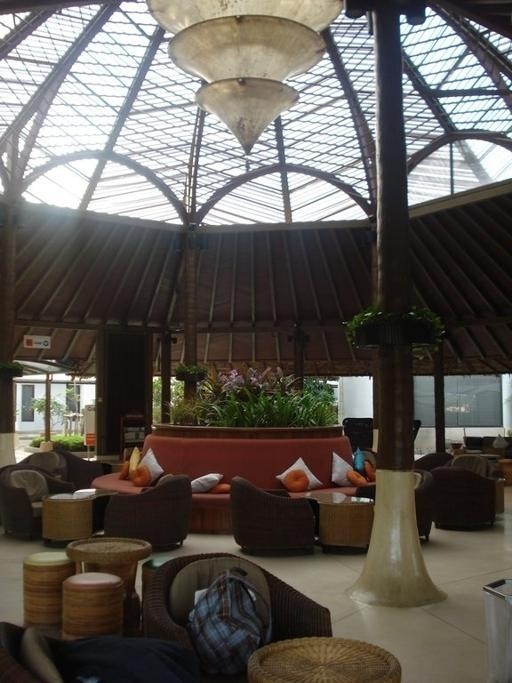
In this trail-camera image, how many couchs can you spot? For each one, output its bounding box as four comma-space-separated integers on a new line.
19, 451, 110, 486
94, 435, 365, 535
232, 476, 318, 554
358, 471, 431, 544
0, 624, 203, 683
450, 454, 504, 511
142, 552, 332, 683
464, 436, 511, 455
0, 464, 74, 540
105, 476, 192, 549
434, 469, 495, 528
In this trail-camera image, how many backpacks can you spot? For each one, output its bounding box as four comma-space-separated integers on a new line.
191, 570, 272, 674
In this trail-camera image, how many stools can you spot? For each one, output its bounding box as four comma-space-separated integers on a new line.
63, 573, 124, 640
24, 552, 82, 631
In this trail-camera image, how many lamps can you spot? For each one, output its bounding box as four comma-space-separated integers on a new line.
148, 1, 341, 154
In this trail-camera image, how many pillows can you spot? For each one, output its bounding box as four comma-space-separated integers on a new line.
119, 460, 129, 478
364, 463, 374, 482
331, 452, 355, 489
136, 449, 162, 482
276, 458, 325, 490
355, 451, 377, 474
127, 450, 139, 477
284, 470, 309, 492
132, 465, 150, 486
189, 472, 223, 493
493, 434, 509, 449
347, 470, 367, 485
211, 483, 231, 493
